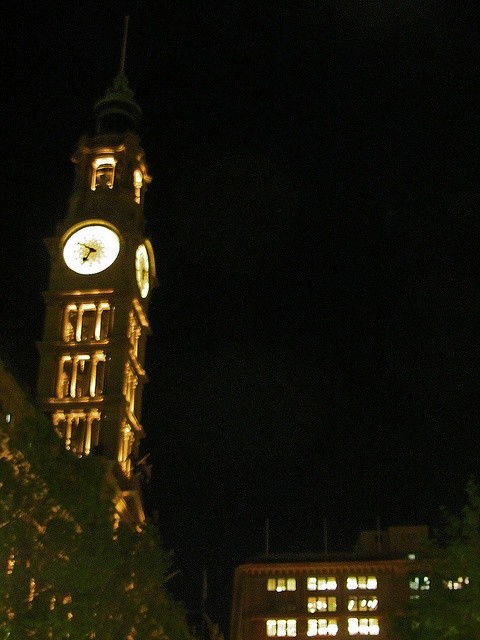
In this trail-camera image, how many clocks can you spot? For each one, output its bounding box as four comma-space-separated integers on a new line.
135, 239, 157, 300
58, 218, 123, 276
